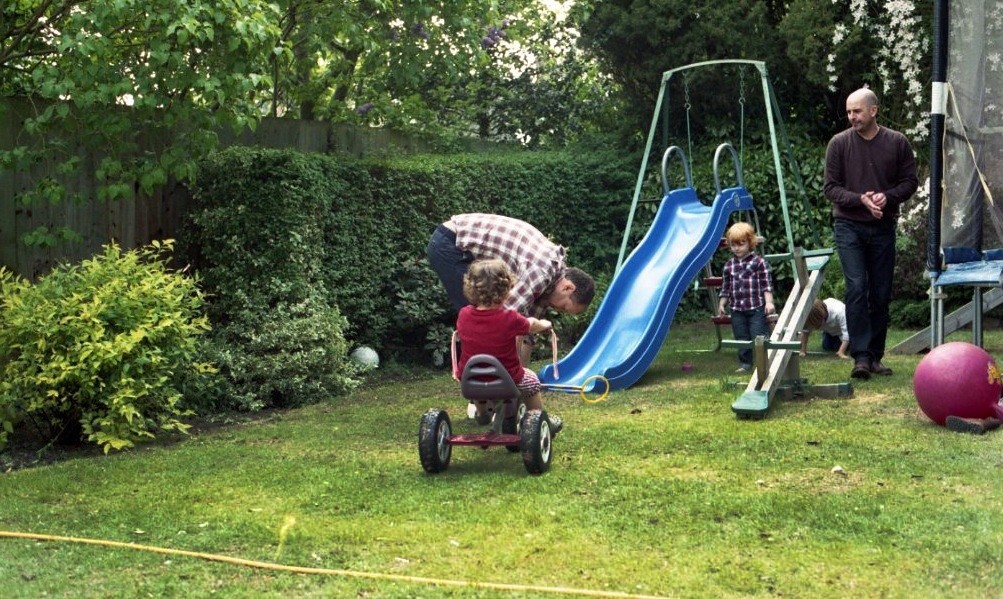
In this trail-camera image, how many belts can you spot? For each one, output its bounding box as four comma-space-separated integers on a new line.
437, 224, 457, 240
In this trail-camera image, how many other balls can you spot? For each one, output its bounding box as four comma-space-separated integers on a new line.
913, 341, 1003, 426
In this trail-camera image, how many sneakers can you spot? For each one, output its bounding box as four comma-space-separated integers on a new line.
851, 357, 871, 379
869, 360, 893, 375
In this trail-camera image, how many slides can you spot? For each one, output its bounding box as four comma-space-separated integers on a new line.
541, 188, 754, 393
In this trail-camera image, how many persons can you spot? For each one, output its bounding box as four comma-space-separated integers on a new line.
426, 213, 595, 419
803, 297, 849, 358
457, 258, 562, 434
946, 391, 1003, 435
718, 222, 775, 373
823, 88, 919, 378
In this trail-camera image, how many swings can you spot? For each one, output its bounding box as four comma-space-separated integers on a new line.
682, 70, 746, 291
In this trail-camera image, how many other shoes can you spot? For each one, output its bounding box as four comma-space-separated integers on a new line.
476, 403, 493, 425
548, 414, 562, 432
467, 402, 495, 422
736, 367, 745, 373
